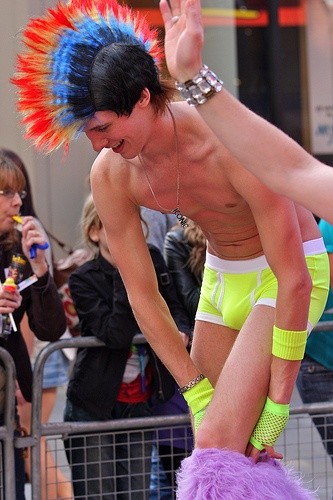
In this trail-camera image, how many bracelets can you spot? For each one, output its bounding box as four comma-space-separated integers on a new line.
178, 374, 205, 395
176, 64, 223, 107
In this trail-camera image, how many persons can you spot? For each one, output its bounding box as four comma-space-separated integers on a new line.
12, 0, 333, 460
159, 0, 333, 225
0, 147, 207, 500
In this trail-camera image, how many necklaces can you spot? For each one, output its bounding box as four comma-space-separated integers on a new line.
137, 103, 190, 229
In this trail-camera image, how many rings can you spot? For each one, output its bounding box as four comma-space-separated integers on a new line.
172, 16, 179, 22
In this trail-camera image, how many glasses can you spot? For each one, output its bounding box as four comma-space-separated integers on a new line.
0, 189, 27, 199
